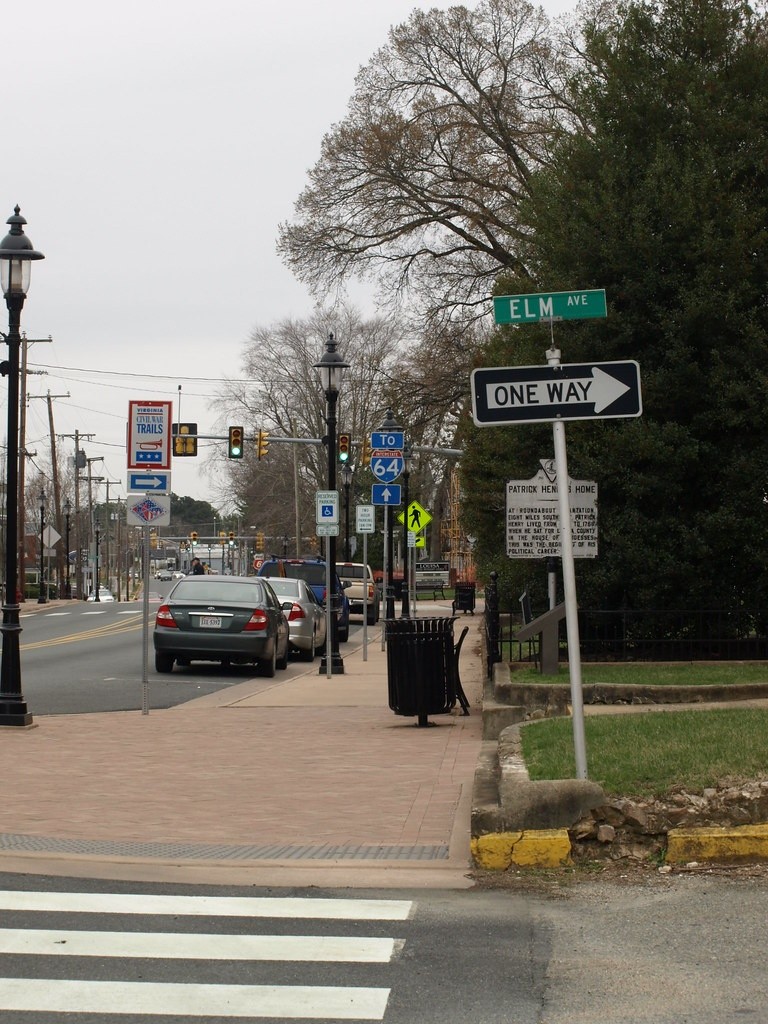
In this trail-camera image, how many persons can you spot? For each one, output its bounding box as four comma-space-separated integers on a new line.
192, 557, 205, 575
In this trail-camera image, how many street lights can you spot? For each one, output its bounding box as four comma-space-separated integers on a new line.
0, 199, 45, 731
36, 487, 47, 603
375, 409, 404, 641
94, 518, 101, 601
283, 533, 288, 558
312, 330, 346, 676
401, 443, 412, 621
125, 526, 143, 601
338, 465, 355, 562
63, 498, 72, 599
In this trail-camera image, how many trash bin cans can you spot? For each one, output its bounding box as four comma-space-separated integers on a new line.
383, 615, 462, 725
392, 579, 405, 601
452, 582, 478, 614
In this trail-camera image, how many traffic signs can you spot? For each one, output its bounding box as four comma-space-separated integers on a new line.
356, 504, 376, 534
469, 358, 644, 428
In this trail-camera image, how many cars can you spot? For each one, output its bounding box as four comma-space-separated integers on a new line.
86, 588, 115, 603
250, 575, 326, 662
154, 568, 186, 582
153, 574, 294, 678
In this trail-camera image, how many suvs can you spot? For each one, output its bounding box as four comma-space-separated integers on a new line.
255, 553, 352, 643
334, 561, 383, 626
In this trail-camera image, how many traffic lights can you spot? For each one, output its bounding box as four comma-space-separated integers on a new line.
337, 432, 351, 464
361, 437, 372, 464
257, 428, 270, 461
192, 532, 197, 546
229, 532, 234, 545
256, 532, 265, 552
228, 426, 244, 458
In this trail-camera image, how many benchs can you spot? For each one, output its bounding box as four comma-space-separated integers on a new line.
454, 626, 470, 715
433, 581, 446, 602
